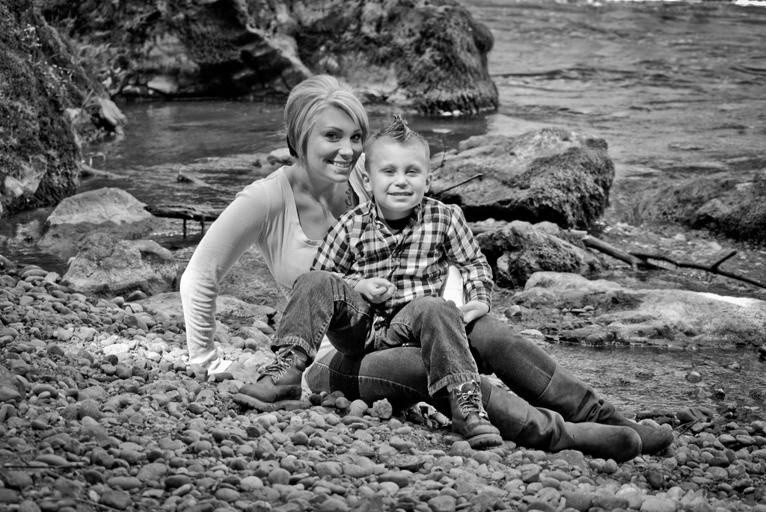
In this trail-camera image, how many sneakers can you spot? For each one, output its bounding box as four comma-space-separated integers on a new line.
449, 380, 505, 450
233, 351, 306, 412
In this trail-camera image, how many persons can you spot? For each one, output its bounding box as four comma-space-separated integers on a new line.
235, 111, 506, 449
177, 72, 675, 462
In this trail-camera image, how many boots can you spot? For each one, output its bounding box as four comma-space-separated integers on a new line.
537, 358, 675, 455
484, 381, 644, 462
